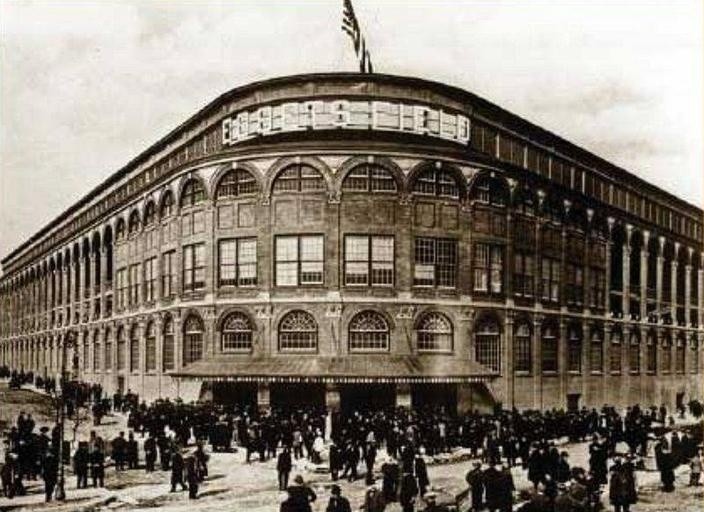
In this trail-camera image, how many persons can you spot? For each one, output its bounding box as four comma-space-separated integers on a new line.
0, 365, 703, 510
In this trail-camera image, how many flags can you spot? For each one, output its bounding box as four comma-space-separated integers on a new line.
340, 0, 371, 74
